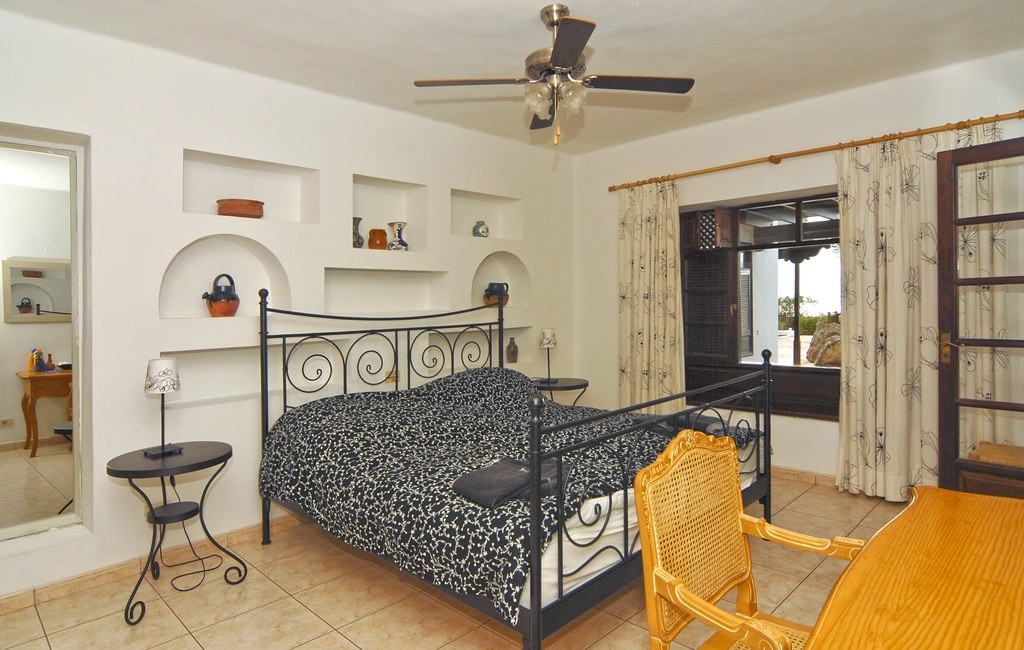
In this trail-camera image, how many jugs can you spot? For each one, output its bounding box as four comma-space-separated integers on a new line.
16, 297, 32, 313
203, 273, 239, 316
483, 281, 509, 308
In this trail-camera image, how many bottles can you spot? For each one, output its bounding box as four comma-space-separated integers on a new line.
507, 336, 518, 363
28, 348, 54, 373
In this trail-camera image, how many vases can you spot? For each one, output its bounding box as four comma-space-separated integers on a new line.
506, 337, 518, 364
368, 229, 388, 250
386, 221, 409, 251
352, 217, 366, 249
472, 221, 488, 238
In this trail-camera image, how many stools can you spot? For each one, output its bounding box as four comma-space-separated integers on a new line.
953, 441, 1024, 498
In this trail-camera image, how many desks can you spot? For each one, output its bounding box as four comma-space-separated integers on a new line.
106, 438, 250, 626
529, 376, 589, 406
16, 362, 73, 461
808, 481, 1024, 649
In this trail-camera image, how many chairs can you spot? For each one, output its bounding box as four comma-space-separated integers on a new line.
632, 428, 868, 649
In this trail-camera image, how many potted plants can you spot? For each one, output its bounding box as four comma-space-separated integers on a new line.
218, 199, 265, 219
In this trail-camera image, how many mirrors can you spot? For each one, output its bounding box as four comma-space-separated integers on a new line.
0, 122, 99, 550
0, 256, 71, 325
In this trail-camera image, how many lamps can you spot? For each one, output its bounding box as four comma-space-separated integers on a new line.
140, 356, 182, 456
539, 325, 558, 384
522, 3, 587, 144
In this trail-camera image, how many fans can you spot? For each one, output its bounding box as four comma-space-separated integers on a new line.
411, 1, 696, 130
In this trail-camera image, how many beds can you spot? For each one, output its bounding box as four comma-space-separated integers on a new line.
256, 283, 775, 649
